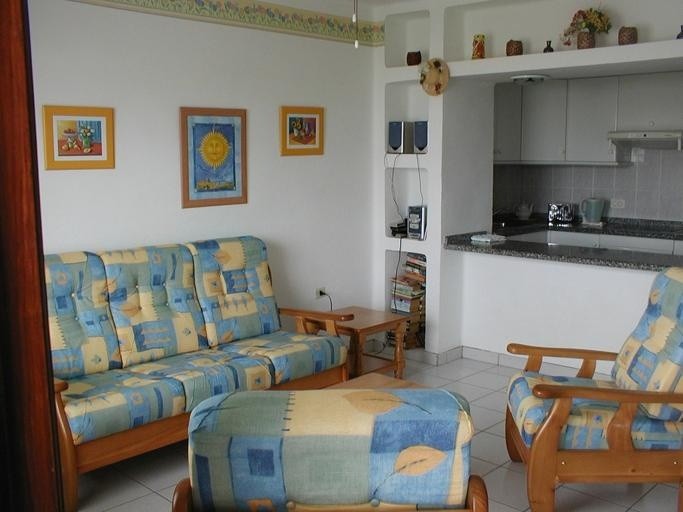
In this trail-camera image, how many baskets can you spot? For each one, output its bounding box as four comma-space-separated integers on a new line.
506, 40, 523, 56
577, 32, 595, 50
618, 26, 637, 45
407, 51, 421, 66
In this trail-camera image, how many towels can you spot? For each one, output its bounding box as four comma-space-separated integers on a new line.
472, 233, 506, 242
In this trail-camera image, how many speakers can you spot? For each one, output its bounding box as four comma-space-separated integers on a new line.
414, 120, 429, 154
389, 122, 413, 154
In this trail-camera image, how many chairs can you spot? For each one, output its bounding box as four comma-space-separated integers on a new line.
505, 266, 683, 512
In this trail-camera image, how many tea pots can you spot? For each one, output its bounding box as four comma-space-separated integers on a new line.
512, 201, 534, 219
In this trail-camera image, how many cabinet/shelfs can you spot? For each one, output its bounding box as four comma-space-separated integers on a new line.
566, 76, 634, 168
494, 82, 522, 166
443, 211, 683, 375
607, 71, 683, 152
520, 78, 566, 169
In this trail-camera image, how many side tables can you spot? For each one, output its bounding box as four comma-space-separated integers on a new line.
296, 304, 412, 380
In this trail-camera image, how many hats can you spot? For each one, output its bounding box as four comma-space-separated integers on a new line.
420, 58, 449, 96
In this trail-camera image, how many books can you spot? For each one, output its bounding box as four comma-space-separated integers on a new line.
399, 253, 426, 282
390, 275, 425, 299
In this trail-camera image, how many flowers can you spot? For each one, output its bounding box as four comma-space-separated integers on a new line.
564, 7, 611, 45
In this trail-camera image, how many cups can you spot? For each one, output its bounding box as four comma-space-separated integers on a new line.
581, 198, 604, 223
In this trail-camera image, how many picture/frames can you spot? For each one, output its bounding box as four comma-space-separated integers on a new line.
180, 107, 248, 210
279, 105, 324, 156
42, 104, 116, 171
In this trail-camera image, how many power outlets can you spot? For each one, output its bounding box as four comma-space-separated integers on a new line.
315, 287, 326, 299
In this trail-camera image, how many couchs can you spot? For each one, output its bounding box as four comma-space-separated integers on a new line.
42, 235, 355, 512
171, 389, 489, 511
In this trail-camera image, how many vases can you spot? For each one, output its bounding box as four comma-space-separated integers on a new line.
543, 40, 554, 53
